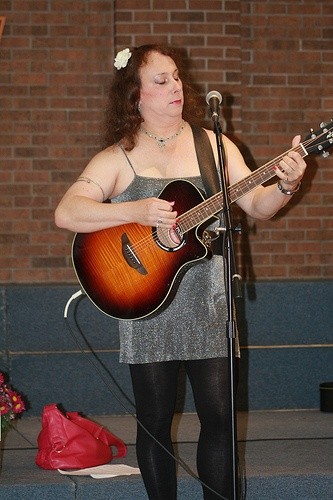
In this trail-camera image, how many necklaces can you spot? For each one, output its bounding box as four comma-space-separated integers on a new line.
137, 120, 186, 148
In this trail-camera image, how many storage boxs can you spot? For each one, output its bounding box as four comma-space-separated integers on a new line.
319, 382, 333, 413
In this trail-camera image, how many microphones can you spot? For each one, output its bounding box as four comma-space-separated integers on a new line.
206, 90, 222, 123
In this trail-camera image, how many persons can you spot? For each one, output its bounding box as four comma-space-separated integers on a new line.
54, 44, 307, 500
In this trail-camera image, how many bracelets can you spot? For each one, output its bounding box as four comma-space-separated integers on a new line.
277, 179, 301, 196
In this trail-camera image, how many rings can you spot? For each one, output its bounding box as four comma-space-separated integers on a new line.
156, 223, 159, 229
158, 217, 164, 224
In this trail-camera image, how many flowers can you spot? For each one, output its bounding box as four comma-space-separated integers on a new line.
113, 46, 132, 70
0, 374, 26, 430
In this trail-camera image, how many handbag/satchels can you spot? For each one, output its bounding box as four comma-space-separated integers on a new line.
36, 404, 128, 470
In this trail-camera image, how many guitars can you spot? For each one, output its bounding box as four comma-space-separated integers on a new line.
69, 117, 332, 321
